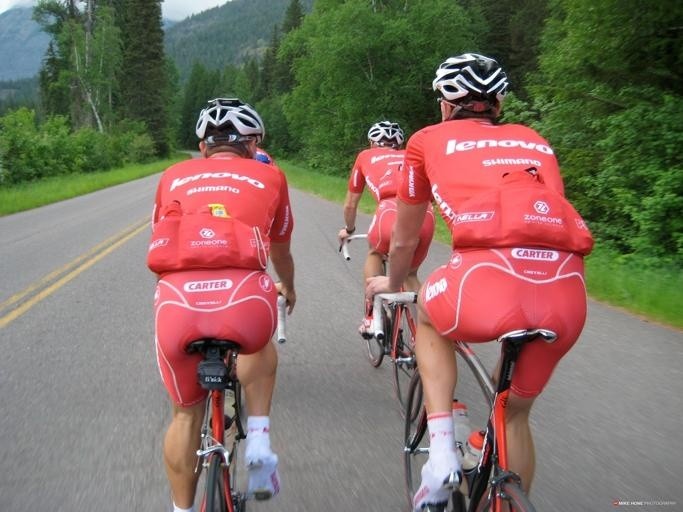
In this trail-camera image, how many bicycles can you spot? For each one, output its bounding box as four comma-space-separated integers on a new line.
186, 293, 286, 512
340, 228, 424, 418
360, 275, 560, 512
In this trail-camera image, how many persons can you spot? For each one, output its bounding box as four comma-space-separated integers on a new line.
360, 49, 596, 507
144, 95, 297, 512
337, 118, 439, 332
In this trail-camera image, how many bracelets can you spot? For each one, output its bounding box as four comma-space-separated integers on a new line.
345, 225, 356, 234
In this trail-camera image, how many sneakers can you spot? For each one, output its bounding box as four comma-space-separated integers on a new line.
246, 440, 280, 499
413, 452, 463, 512
358, 317, 376, 335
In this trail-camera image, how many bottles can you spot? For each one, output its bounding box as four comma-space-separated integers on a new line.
448, 402, 471, 447
460, 428, 489, 471
208, 413, 233, 458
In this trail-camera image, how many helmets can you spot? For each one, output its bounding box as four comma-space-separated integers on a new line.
432, 53, 510, 111
367, 121, 404, 148
195, 98, 264, 144
